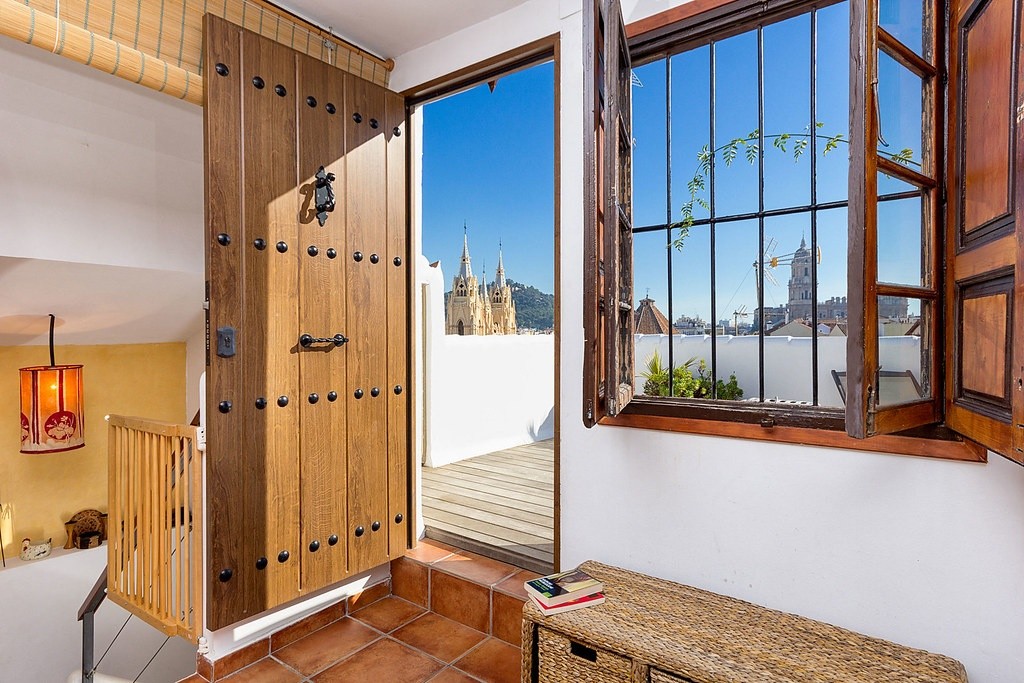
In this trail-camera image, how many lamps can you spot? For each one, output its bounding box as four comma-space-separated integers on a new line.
19, 365, 85, 456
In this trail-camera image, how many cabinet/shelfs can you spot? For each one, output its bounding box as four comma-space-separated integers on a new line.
521, 558, 968, 683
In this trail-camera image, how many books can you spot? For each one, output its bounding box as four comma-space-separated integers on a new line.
524, 569, 605, 617
